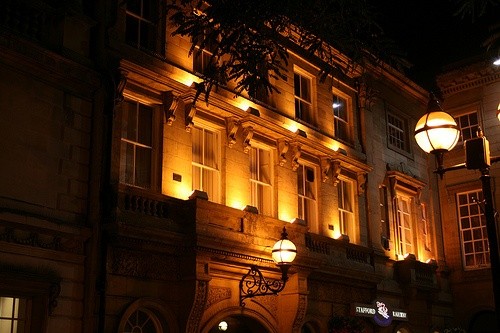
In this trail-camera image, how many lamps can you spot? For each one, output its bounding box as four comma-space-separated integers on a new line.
239, 226, 296, 308
